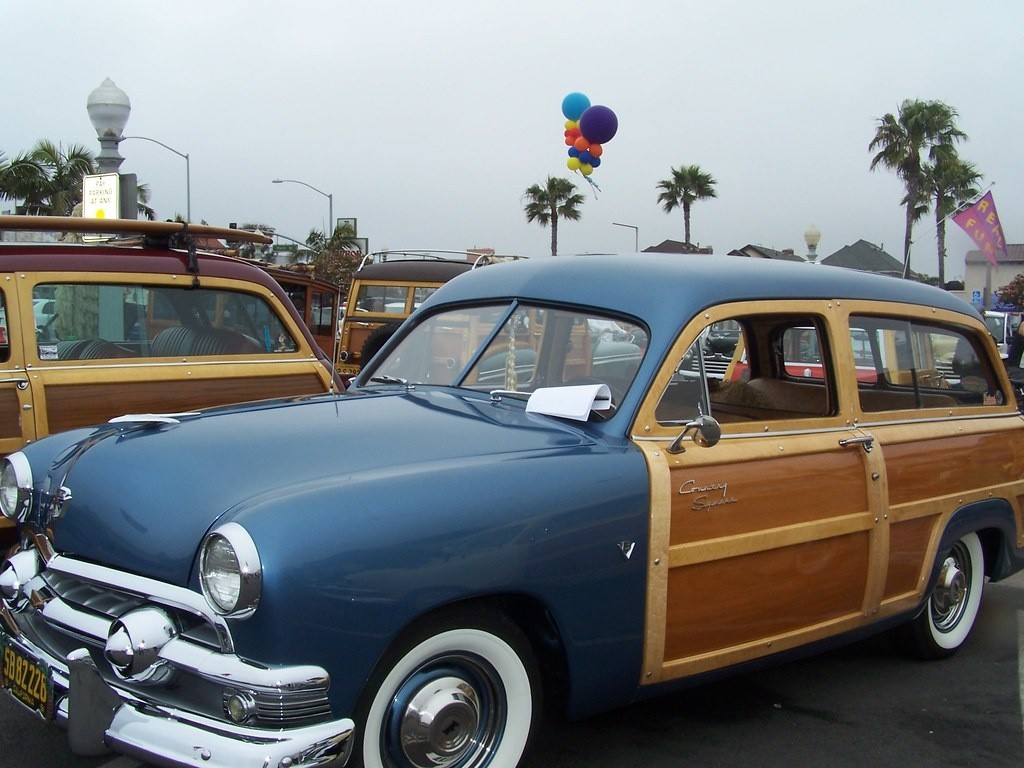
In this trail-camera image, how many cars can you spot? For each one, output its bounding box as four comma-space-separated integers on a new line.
0, 298, 59, 343
930, 333, 961, 386
1, 213, 347, 533
146, 256, 348, 364
723, 324, 951, 390
678, 316, 748, 379
1, 251, 1023, 767
585, 318, 642, 379
985, 310, 1013, 361
335, 250, 594, 395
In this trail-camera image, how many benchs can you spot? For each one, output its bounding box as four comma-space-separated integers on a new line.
54, 326, 264, 361
595, 375, 959, 428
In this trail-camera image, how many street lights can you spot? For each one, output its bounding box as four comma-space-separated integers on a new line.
119, 136, 191, 223
271, 180, 333, 238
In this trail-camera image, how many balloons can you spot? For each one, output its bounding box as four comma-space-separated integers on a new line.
562, 92, 618, 176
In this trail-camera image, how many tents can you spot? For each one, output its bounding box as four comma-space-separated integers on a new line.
970, 293, 1015, 312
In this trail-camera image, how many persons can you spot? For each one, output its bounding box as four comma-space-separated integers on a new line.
952, 305, 997, 385
